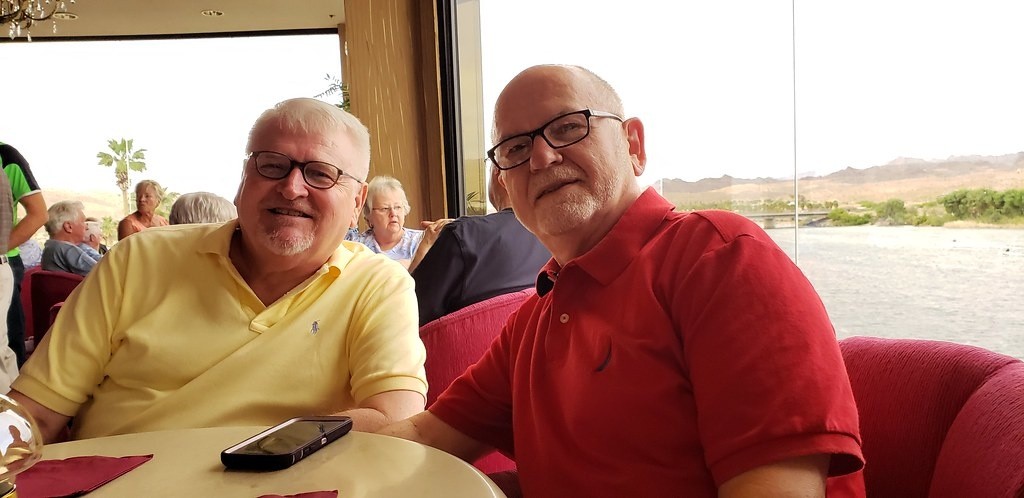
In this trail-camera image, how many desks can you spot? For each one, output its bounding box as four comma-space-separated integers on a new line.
0, 426, 507, 498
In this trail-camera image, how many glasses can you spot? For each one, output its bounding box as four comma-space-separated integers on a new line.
244, 149, 367, 190
370, 205, 406, 214
482, 109, 626, 173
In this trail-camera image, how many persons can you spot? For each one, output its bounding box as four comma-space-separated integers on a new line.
76, 220, 104, 262
168, 188, 239, 227
0, 161, 21, 399
0, 137, 49, 373
1, 96, 431, 440
404, 149, 561, 327
118, 181, 171, 241
368, 63, 868, 498
344, 175, 427, 265
40, 200, 99, 278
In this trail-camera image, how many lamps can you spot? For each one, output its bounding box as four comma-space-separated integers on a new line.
0, 0, 79, 43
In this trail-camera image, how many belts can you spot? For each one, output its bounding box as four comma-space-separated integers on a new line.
0, 254, 10, 266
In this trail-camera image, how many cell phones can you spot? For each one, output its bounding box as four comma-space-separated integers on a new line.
220, 414, 353, 469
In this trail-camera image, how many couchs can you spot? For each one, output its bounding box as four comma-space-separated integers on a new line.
408, 285, 547, 476
30, 271, 89, 352
489, 334, 1022, 498
19, 265, 46, 341
